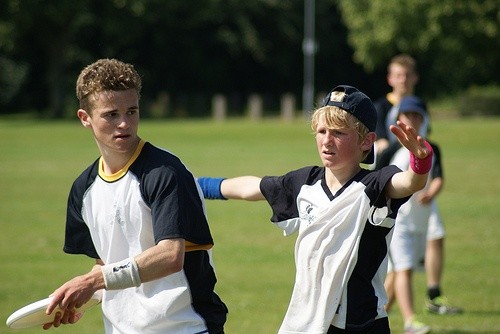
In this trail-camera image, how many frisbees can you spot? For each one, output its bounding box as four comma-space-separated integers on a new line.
6, 293, 101, 332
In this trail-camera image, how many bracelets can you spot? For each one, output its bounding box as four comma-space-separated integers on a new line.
101, 257, 141, 290
410, 140, 434, 174
196, 176, 228, 200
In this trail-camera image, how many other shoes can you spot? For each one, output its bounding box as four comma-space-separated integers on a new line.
426, 297, 464, 316
402, 317, 432, 334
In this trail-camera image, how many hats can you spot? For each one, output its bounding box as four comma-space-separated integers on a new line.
320, 84, 378, 164
400, 94, 428, 117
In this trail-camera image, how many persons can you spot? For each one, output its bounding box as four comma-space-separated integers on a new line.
196, 85, 434, 334
42, 59, 229, 334
371, 53, 464, 315
374, 95, 443, 334
